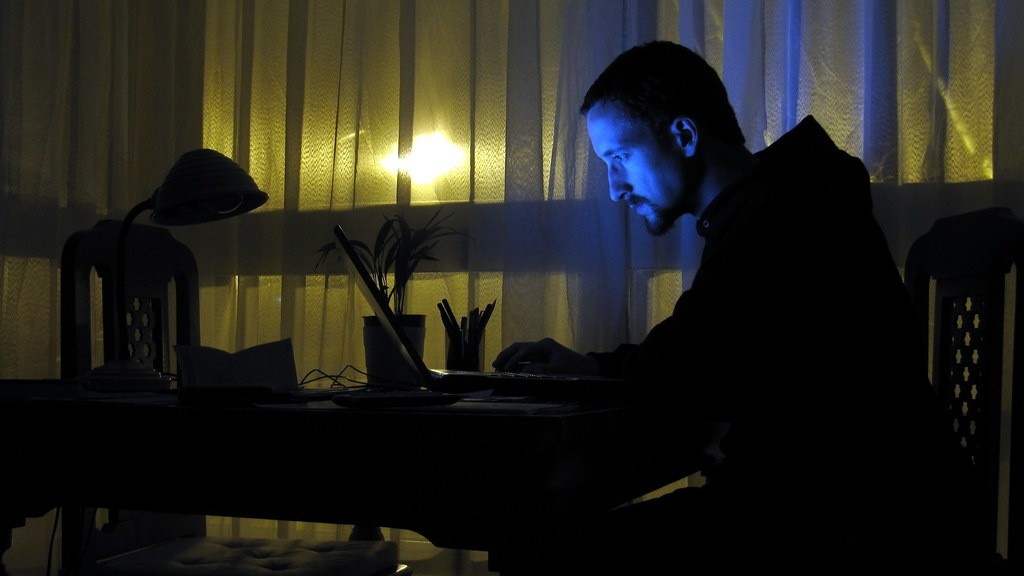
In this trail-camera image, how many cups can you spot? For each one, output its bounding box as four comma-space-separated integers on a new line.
445, 329, 486, 374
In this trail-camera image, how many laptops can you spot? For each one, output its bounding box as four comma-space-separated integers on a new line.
332, 224, 640, 399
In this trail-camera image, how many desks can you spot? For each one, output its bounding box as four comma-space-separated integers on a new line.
2, 378, 712, 576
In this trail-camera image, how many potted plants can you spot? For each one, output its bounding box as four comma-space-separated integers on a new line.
311, 204, 476, 392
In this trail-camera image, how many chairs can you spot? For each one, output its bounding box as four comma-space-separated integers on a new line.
902, 207, 1023, 575
59, 219, 418, 576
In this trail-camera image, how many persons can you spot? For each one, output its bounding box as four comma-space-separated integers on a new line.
490, 38, 941, 573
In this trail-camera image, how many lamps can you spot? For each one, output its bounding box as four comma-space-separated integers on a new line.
82, 149, 272, 396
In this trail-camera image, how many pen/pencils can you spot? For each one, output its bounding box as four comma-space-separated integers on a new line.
436, 297, 497, 369
